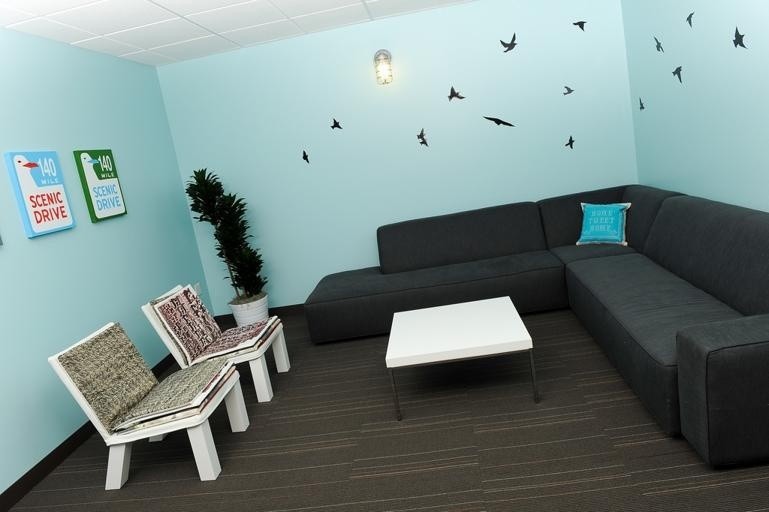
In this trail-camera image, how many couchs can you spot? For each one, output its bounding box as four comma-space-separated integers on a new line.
301, 176, 769, 466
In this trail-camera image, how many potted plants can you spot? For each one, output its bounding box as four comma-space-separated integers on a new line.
184, 166, 270, 327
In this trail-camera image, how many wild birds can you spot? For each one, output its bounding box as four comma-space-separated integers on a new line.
639, 97, 645, 111
417, 128, 426, 140
448, 86, 464, 101
652, 35, 664, 54
302, 150, 310, 163
330, 118, 342, 130
731, 27, 748, 50
420, 140, 429, 147
482, 115, 516, 128
573, 20, 587, 32
565, 135, 575, 149
563, 86, 574, 96
499, 32, 517, 52
685, 12, 695, 27
671, 65, 684, 86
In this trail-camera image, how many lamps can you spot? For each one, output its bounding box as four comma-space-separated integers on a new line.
373, 48, 393, 85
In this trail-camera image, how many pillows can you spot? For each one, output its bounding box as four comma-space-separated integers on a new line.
575, 201, 633, 246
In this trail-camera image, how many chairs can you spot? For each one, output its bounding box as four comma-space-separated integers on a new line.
140, 283, 291, 404
47, 321, 251, 492
